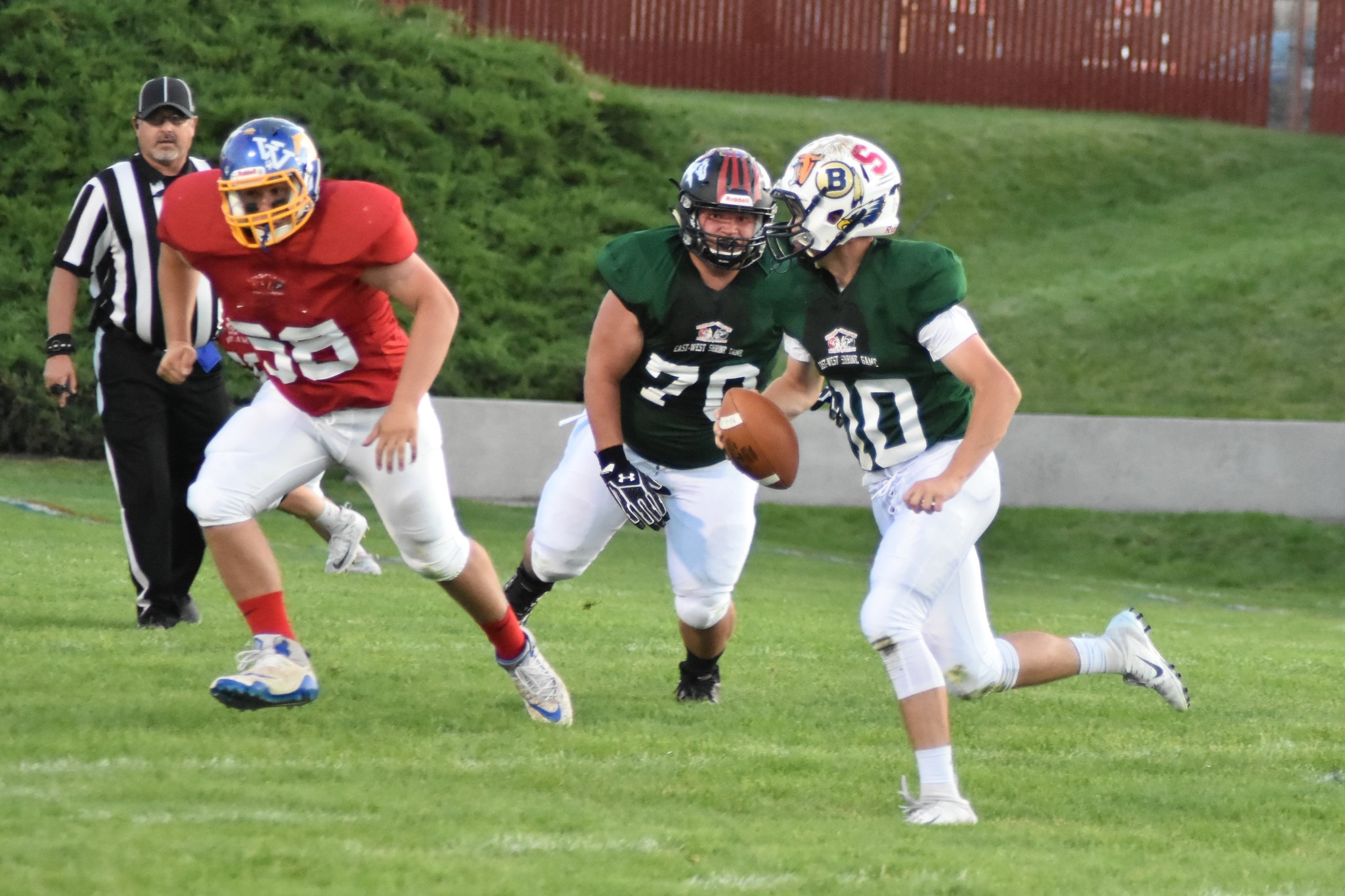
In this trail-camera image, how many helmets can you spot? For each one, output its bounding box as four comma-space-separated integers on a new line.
768, 133, 902, 252
218, 116, 323, 249
672, 147, 776, 271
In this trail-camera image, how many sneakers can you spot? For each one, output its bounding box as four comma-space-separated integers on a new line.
1109, 609, 1190, 712
323, 501, 368, 577
898, 778, 977, 827
346, 554, 383, 575
675, 661, 723, 707
502, 565, 548, 625
208, 634, 319, 713
493, 626, 572, 730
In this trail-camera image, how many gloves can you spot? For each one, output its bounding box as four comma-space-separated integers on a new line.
600, 459, 675, 533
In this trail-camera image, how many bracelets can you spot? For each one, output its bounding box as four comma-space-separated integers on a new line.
37, 340, 74, 351
45, 333, 74, 359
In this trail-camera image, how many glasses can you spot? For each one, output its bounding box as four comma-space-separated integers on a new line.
136, 113, 188, 126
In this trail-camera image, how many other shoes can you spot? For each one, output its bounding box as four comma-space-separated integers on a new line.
180, 600, 203, 626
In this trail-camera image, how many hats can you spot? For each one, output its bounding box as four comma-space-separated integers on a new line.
135, 76, 194, 118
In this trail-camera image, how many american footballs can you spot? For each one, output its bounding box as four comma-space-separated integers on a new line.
718, 387, 799, 490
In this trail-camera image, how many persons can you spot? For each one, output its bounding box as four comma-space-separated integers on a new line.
215, 308, 382, 574
501, 148, 825, 702
152, 114, 573, 726
714, 133, 1191, 825
43, 75, 228, 626
